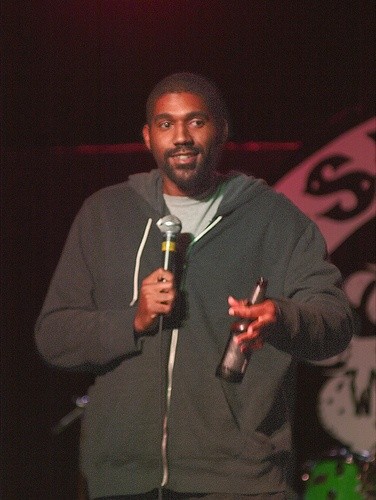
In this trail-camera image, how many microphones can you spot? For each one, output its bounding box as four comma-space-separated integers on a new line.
161, 216, 182, 292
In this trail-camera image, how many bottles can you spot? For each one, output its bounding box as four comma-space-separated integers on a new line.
214, 276, 269, 384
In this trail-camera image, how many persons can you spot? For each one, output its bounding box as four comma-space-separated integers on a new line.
34, 72, 355, 500
